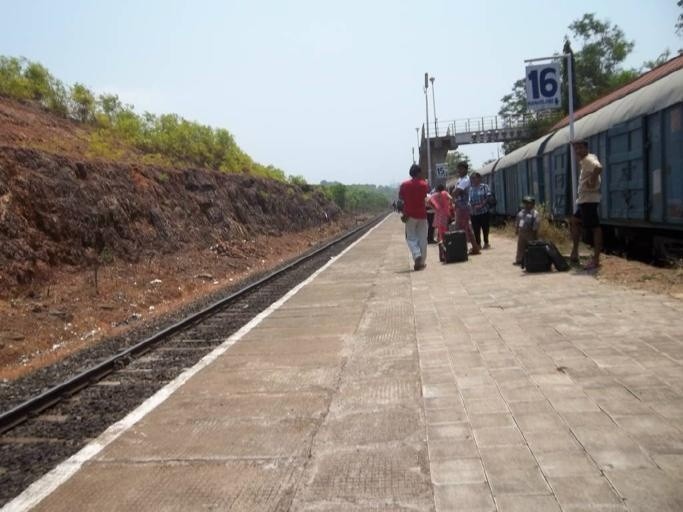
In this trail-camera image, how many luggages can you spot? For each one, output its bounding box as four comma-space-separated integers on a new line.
526, 239, 550, 272
444, 230, 468, 264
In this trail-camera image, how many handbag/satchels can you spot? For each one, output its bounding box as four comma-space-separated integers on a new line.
546, 241, 569, 271
488, 195, 497, 206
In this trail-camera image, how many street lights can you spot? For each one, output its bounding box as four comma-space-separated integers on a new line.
423, 72, 431, 187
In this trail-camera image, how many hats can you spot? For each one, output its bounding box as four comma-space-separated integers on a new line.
522, 196, 536, 204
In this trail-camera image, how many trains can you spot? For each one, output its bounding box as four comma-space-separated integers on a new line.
466, 54, 683, 234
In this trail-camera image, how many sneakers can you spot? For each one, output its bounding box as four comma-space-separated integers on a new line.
414, 256, 426, 271
469, 243, 490, 255
569, 257, 600, 270
513, 262, 523, 266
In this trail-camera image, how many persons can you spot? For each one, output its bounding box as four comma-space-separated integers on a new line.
450, 161, 482, 255
466, 172, 495, 249
398, 164, 429, 271
391, 183, 455, 246
511, 196, 539, 266
566, 139, 604, 270
